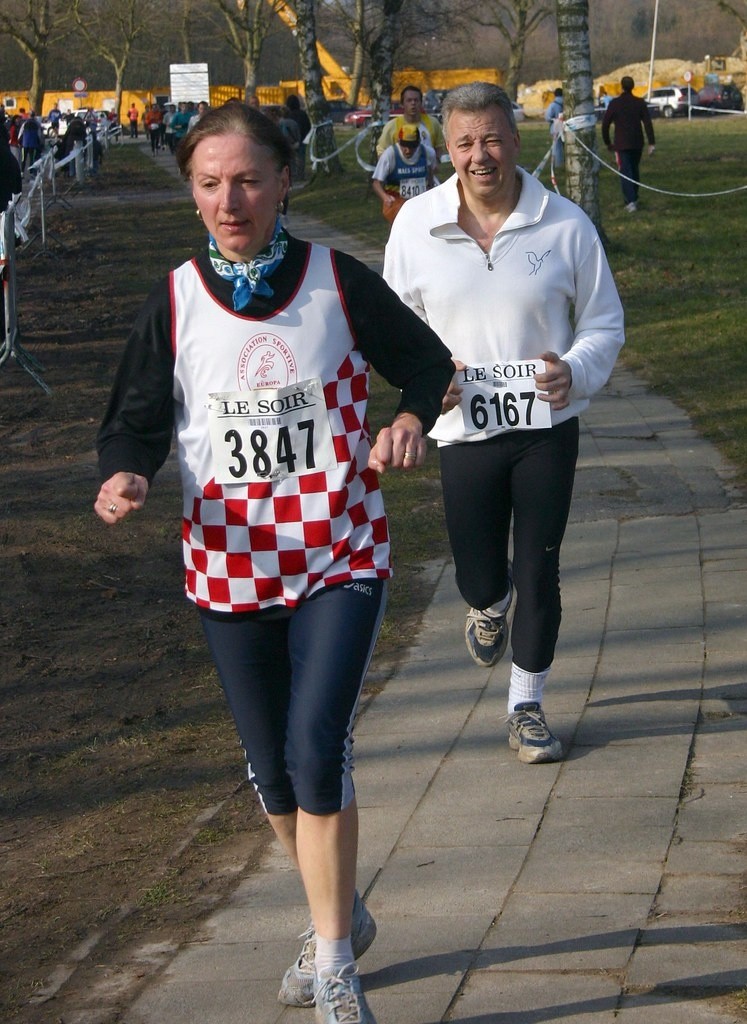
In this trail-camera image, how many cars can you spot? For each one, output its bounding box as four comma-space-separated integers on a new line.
510, 101, 525, 122
256, 104, 284, 121
327, 99, 361, 125
342, 100, 430, 127
425, 89, 447, 117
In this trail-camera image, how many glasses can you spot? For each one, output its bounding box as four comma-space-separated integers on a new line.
398, 140, 420, 147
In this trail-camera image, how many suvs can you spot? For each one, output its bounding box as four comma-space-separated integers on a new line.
35, 109, 112, 137
642, 87, 702, 118
694, 79, 744, 115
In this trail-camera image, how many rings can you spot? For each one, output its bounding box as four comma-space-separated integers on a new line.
404, 452, 416, 460
108, 503, 118, 513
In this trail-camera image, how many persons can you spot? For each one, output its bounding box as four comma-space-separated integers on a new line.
602, 76, 656, 213
278, 95, 311, 183
372, 86, 443, 223
382, 82, 626, 764
94, 97, 456, 1024
546, 88, 564, 169
0, 104, 98, 251
601, 92, 613, 109
127, 103, 138, 138
249, 95, 260, 111
141, 101, 209, 181
108, 108, 119, 144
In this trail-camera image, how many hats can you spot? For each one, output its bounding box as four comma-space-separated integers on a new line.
397, 124, 420, 141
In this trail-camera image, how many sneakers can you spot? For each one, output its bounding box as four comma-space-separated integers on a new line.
311, 959, 377, 1024
277, 888, 377, 1008
464, 558, 513, 668
502, 701, 563, 763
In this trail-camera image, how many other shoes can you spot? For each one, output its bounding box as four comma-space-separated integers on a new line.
623, 201, 637, 213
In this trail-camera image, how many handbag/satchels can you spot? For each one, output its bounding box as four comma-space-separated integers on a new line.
285, 127, 299, 150
